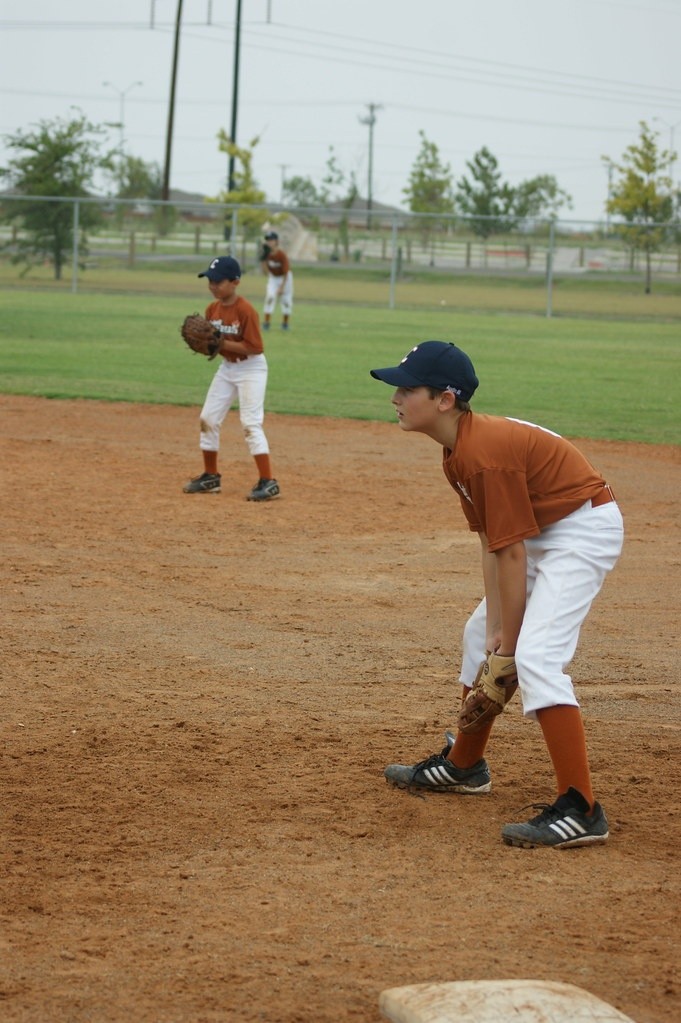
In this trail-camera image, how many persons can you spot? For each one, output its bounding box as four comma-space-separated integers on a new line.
370, 340, 624, 846
180, 255, 280, 501
257, 232, 292, 329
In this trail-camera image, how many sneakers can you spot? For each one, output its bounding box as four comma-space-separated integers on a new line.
384, 730, 492, 793
263, 323, 269, 330
283, 323, 288, 329
248, 478, 280, 501
501, 785, 610, 849
183, 471, 221, 494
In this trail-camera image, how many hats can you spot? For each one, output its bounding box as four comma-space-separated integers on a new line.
264, 232, 278, 240
370, 340, 479, 403
198, 255, 241, 282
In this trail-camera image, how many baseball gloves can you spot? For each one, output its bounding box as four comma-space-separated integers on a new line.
260, 243, 271, 260
180, 310, 224, 362
455, 642, 519, 733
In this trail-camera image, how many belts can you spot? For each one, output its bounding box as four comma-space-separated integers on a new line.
591, 485, 616, 508
228, 356, 247, 362
